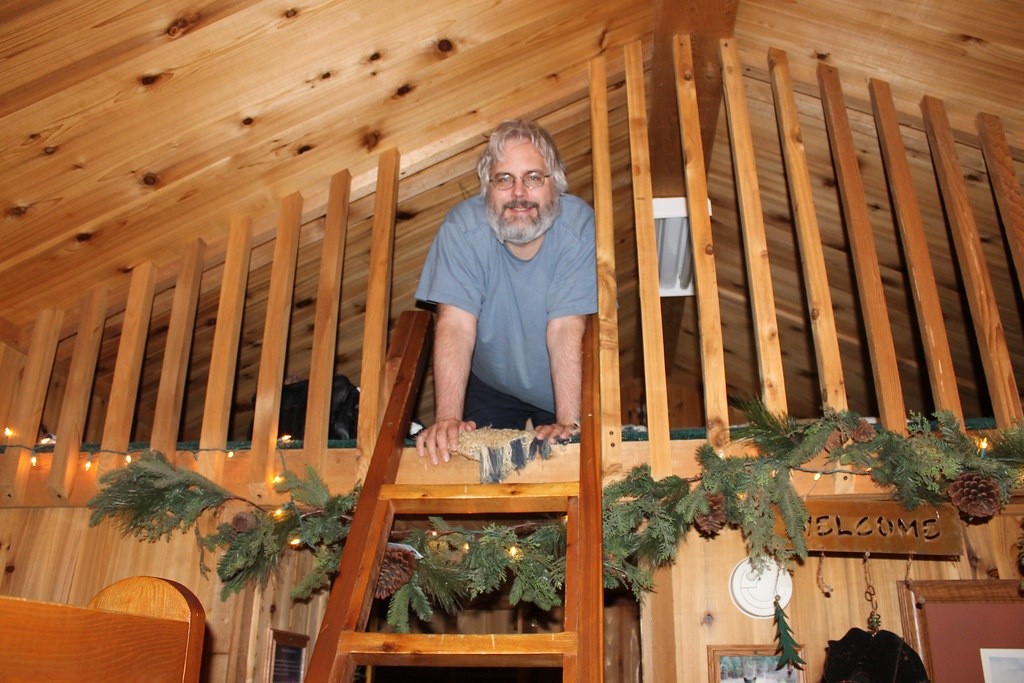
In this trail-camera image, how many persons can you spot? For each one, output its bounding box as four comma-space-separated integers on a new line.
414, 119, 602, 467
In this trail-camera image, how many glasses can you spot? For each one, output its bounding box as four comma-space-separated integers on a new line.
489, 172, 552, 189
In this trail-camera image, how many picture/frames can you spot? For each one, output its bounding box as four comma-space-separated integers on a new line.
265, 628, 311, 683
899, 579, 1024, 683
707, 643, 810, 683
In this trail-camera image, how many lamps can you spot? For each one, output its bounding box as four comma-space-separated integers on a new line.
650, 197, 713, 296
